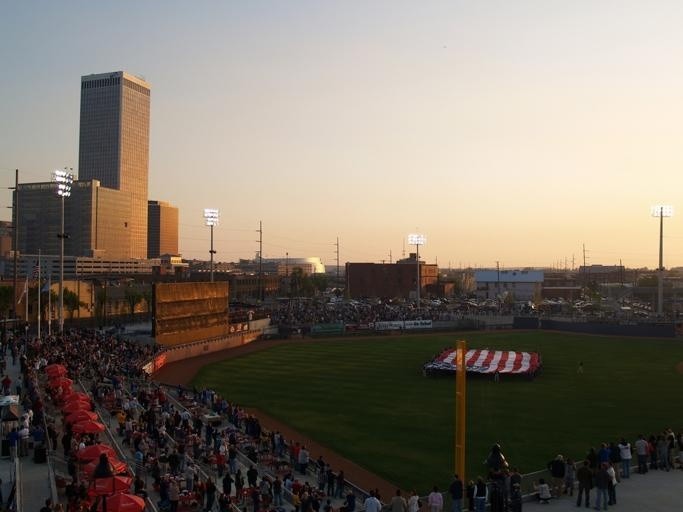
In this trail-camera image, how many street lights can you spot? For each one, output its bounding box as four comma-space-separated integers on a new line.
407, 234, 425, 318
286, 252, 289, 278
651, 204, 673, 315
203, 208, 220, 282
24, 320, 33, 355
52, 169, 73, 332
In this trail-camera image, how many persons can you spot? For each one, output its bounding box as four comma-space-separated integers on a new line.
576, 360, 584, 376
423, 346, 544, 383
229, 294, 683, 325
388, 424, 682, 512
0, 322, 383, 512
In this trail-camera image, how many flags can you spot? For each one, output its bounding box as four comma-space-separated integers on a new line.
16, 281, 26, 304
31, 259, 39, 274
40, 279, 50, 293
